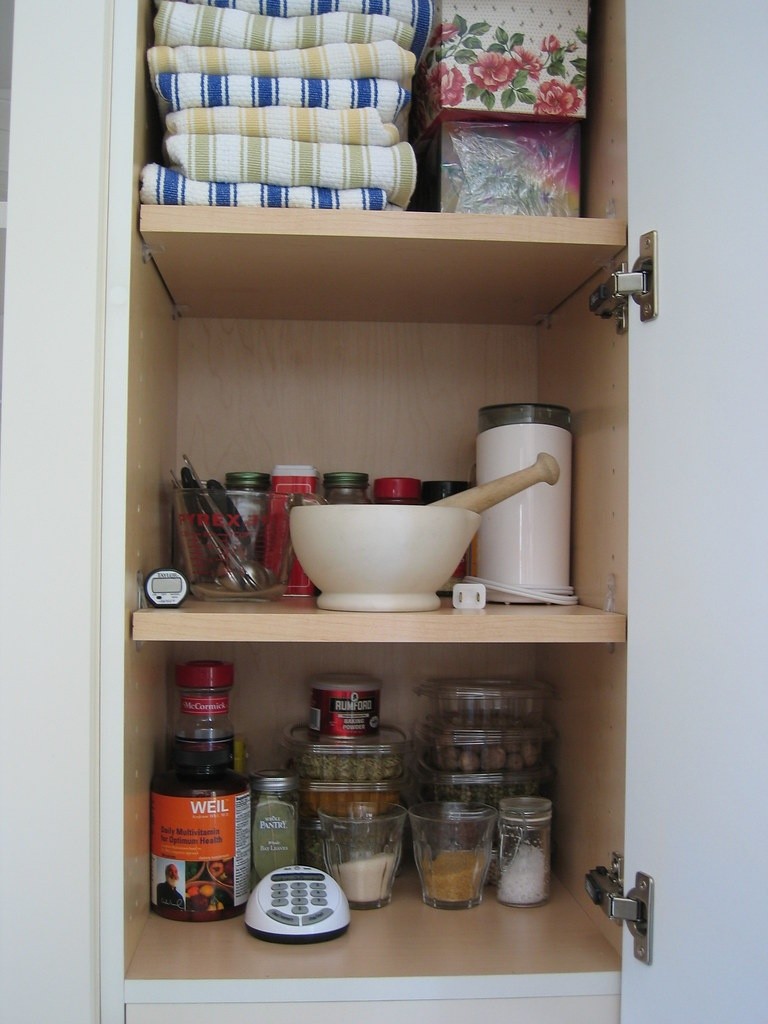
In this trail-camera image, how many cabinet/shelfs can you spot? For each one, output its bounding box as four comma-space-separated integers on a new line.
1, 0, 768, 1024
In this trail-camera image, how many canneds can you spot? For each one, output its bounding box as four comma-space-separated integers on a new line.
306, 672, 382, 740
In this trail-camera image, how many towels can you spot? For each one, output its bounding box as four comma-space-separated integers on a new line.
181, 0, 435, 65
154, 71, 410, 112
164, 106, 400, 147
137, 162, 388, 211
152, 1, 416, 50
147, 39, 417, 91
164, 134, 417, 209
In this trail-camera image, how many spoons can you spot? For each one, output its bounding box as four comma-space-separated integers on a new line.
170, 454, 277, 591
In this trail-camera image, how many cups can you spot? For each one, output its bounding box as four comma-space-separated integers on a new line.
408, 800, 498, 910
166, 488, 329, 602
316, 801, 406, 911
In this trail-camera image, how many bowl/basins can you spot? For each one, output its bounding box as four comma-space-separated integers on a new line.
408, 677, 555, 813
276, 671, 411, 819
288, 504, 481, 612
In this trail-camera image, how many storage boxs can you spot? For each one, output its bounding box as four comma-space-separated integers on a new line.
410, 0, 588, 216
290, 675, 559, 877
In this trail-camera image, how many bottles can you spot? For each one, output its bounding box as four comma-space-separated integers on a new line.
497, 796, 554, 907
147, 659, 302, 924
225, 464, 477, 604
